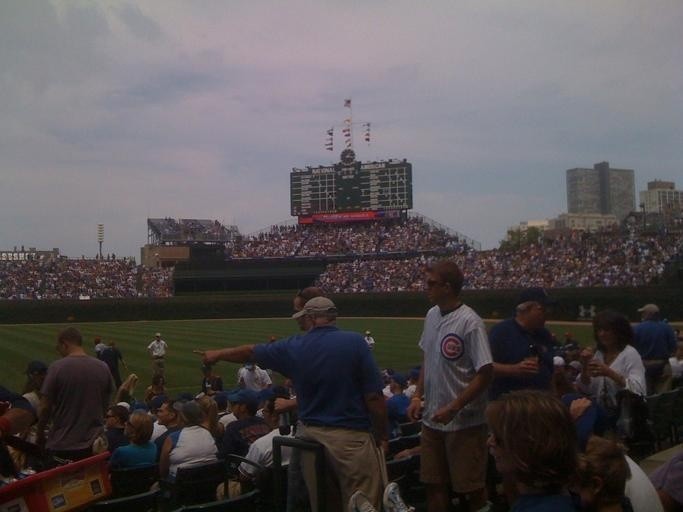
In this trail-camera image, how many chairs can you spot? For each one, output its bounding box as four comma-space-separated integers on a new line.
370, 405, 435, 494
169, 459, 223, 505
654, 389, 678, 448
642, 392, 662, 446
181, 489, 261, 512
110, 461, 159, 492
91, 490, 158, 511
674, 386, 683, 445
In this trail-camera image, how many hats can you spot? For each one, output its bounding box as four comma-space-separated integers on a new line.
294, 287, 326, 312
178, 392, 193, 400
115, 402, 132, 410
152, 394, 164, 407
292, 297, 338, 320
154, 333, 164, 338
637, 304, 660, 313
560, 394, 607, 435
257, 385, 291, 397
515, 286, 559, 305
108, 405, 129, 419
172, 401, 201, 422
226, 389, 259, 410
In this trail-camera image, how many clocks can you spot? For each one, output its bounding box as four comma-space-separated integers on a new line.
340, 149, 357, 166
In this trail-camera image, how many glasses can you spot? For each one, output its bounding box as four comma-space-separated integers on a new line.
262, 410, 268, 414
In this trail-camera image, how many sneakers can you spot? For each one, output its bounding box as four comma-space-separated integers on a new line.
348, 492, 378, 512
383, 483, 415, 512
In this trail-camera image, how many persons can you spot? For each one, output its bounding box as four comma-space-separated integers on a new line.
378, 364, 424, 433
648, 454, 683, 511
290, 287, 326, 331
476, 389, 579, 511
559, 392, 664, 512
496, 217, 683, 286
192, 393, 236, 475
236, 360, 272, 392
223, 389, 273, 477
0, 253, 148, 299
259, 386, 290, 418
631, 305, 677, 403
148, 331, 168, 375
22, 360, 51, 482
549, 331, 591, 394
365, 330, 376, 354
148, 213, 425, 301
254, 390, 266, 420
485, 287, 562, 403
0, 385, 38, 490
575, 311, 655, 455
215, 386, 297, 501
33, 325, 113, 471
99, 364, 228, 512
101, 340, 127, 390
94, 336, 117, 361
193, 296, 390, 512
211, 393, 240, 431
409, 259, 497, 512
346, 434, 634, 511
426, 216, 496, 291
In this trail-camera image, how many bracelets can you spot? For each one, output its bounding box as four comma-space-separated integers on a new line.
411, 397, 420, 401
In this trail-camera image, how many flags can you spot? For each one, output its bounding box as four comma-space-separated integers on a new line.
342, 117, 352, 147
361, 122, 370, 143
324, 129, 334, 151
344, 99, 352, 108
